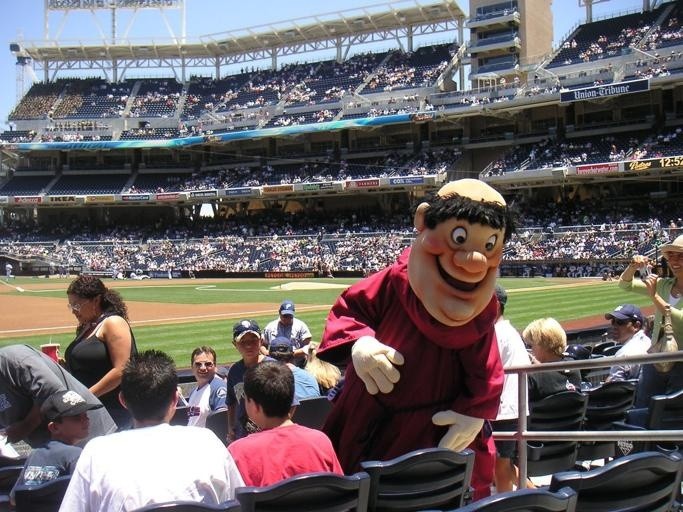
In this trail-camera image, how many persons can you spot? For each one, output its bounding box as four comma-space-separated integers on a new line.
605, 304, 651, 383
9, 391, 103, 512
129, 185, 165, 194
487, 127, 683, 176
504, 190, 683, 275
197, 203, 420, 280
59, 275, 139, 433
259, 301, 311, 359
0, 344, 119, 451
187, 346, 228, 428
5, 261, 15, 283
179, 165, 275, 192
52, 216, 197, 280
55, 349, 247, 512
268, 337, 321, 405
558, 17, 683, 87
179, 59, 346, 139
459, 79, 563, 107
521, 316, 582, 401
0, 76, 179, 144
224, 319, 278, 447
347, 40, 458, 117
0, 219, 49, 256
489, 286, 539, 492
280, 147, 456, 184
227, 360, 345, 489
305, 341, 341, 397
617, 234, 683, 408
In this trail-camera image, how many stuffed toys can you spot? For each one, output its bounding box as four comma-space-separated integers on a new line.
317, 179, 518, 502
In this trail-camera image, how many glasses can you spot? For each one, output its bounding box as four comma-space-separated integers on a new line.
68, 299, 93, 311
611, 317, 634, 326
282, 314, 293, 318
193, 362, 214, 367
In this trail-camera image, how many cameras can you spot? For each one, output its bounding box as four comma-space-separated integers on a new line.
638, 264, 656, 280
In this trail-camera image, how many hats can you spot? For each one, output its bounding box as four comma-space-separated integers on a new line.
495, 283, 508, 304
270, 336, 293, 352
279, 300, 295, 316
658, 234, 683, 261
605, 304, 644, 323
40, 390, 104, 423
232, 319, 261, 343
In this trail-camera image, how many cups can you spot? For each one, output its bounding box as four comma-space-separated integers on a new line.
40, 344, 59, 362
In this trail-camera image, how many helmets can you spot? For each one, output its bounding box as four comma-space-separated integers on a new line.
565, 344, 591, 360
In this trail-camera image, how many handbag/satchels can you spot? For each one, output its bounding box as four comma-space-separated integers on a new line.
646, 335, 679, 373
